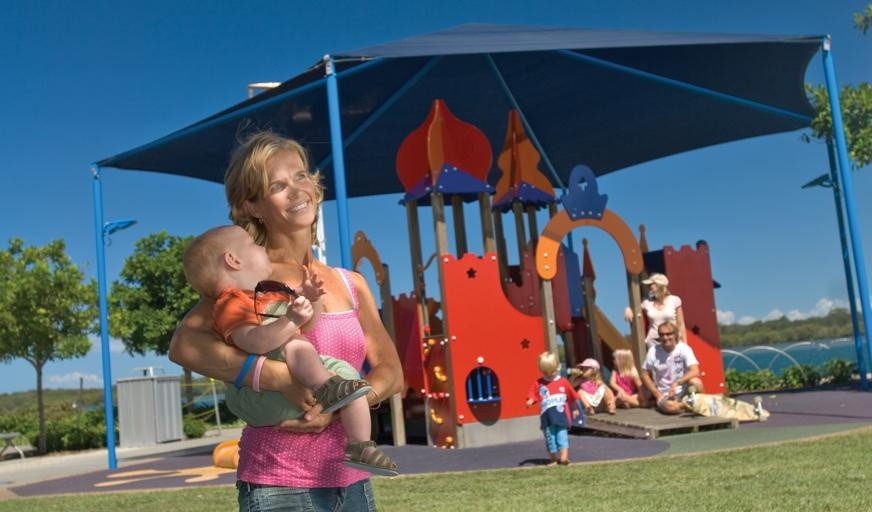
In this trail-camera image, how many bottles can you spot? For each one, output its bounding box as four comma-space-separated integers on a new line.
566, 368, 584, 377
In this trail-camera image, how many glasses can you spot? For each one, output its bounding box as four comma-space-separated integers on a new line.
242, 280, 301, 324
658, 332, 673, 337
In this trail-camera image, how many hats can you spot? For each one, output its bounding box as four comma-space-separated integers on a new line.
642, 273, 668, 286
576, 358, 600, 369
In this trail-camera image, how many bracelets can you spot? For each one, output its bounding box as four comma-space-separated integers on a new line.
369, 387, 381, 411
252, 354, 268, 393
235, 353, 256, 389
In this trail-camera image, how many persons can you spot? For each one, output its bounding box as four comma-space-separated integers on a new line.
608, 348, 649, 409
640, 321, 704, 415
525, 350, 582, 467
168, 128, 404, 512
569, 358, 617, 420
623, 272, 687, 353
183, 223, 400, 479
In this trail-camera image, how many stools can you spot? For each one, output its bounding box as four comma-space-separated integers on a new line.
0, 432, 24, 461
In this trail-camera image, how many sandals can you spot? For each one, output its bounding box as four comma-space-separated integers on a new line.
342, 440, 399, 476
314, 376, 372, 414
